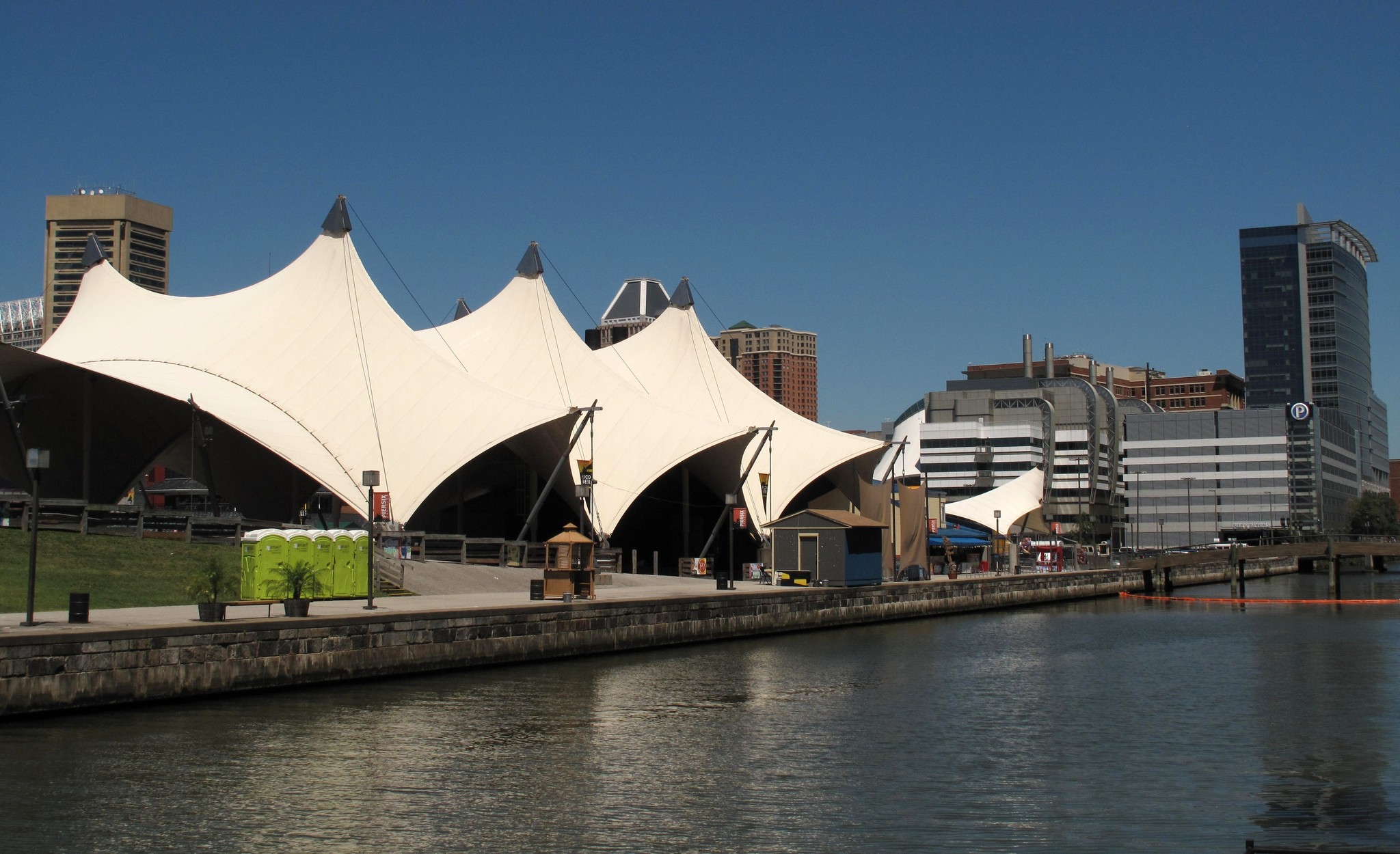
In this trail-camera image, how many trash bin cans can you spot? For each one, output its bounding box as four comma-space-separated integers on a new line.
68, 592, 89, 624
716, 571, 728, 590
530, 579, 544, 601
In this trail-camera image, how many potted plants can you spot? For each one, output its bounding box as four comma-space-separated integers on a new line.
185, 553, 245, 622
261, 560, 332, 617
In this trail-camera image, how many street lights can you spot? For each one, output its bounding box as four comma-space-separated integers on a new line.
1208, 489, 1218, 538
1159, 518, 1164, 553
1180, 477, 1196, 546
993, 510, 1001, 576
574, 484, 588, 568
19, 447, 53, 627
1264, 491, 1274, 545
362, 470, 381, 610
725, 493, 738, 590
1130, 471, 1147, 551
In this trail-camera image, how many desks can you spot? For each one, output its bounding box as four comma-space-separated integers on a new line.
777, 570, 811, 586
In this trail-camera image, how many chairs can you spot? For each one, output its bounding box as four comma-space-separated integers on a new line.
759, 566, 772, 585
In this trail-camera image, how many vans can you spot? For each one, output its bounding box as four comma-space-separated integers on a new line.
1064, 546, 1094, 556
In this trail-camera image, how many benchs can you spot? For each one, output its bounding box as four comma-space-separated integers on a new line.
222, 600, 281, 620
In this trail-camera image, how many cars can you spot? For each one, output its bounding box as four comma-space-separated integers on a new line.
1088, 543, 1248, 568
1002, 545, 1036, 567
